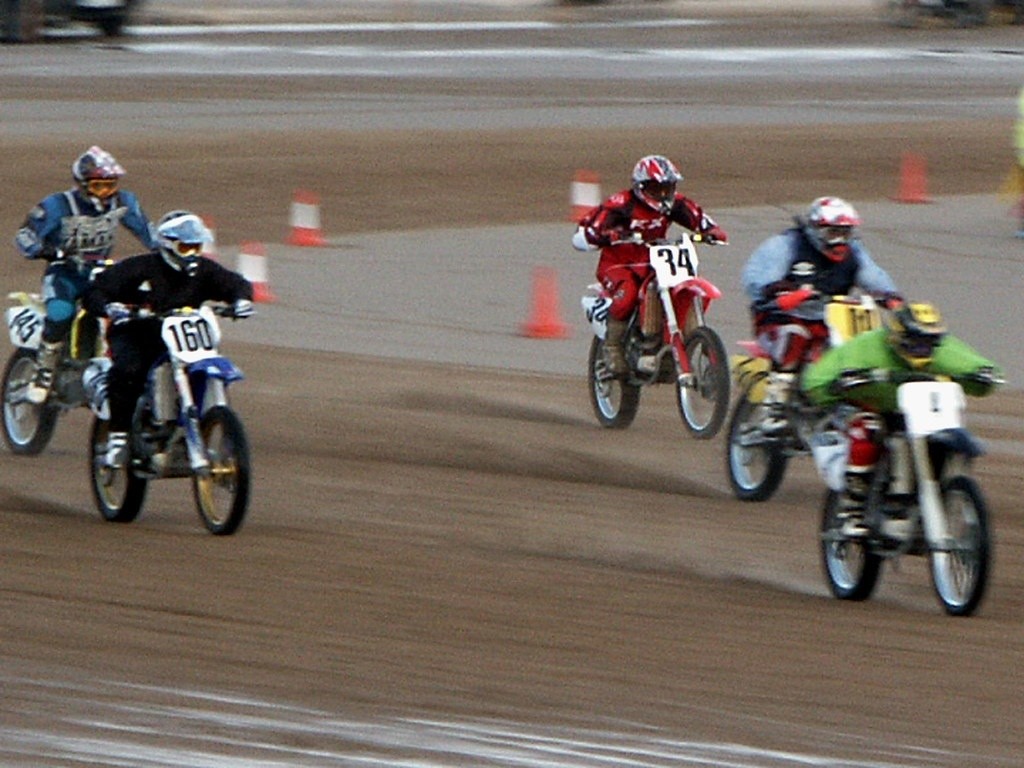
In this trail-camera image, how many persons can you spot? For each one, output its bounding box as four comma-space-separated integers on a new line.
15, 146, 161, 405
81, 209, 255, 469
740, 196, 907, 435
570, 153, 727, 374
798, 302, 1004, 536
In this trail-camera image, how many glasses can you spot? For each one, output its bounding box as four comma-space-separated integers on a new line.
172, 239, 204, 258
820, 226, 854, 246
87, 180, 120, 197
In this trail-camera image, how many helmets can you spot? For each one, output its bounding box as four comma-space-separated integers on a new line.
156, 211, 213, 276
73, 146, 126, 198
890, 301, 948, 368
808, 197, 859, 261
632, 156, 684, 215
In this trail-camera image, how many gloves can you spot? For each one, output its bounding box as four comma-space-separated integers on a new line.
767, 281, 797, 295
600, 231, 617, 244
707, 230, 726, 242
233, 299, 256, 316
37, 246, 56, 262
964, 373, 993, 397
104, 302, 130, 325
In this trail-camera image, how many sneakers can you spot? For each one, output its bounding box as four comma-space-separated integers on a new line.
28, 368, 52, 403
106, 432, 128, 467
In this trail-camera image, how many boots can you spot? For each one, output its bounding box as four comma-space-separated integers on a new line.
760, 372, 796, 431
838, 464, 871, 537
605, 320, 628, 374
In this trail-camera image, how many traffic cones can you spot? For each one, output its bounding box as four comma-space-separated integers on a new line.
888, 151, 934, 203
567, 170, 602, 220
234, 240, 271, 304
520, 266, 567, 338
286, 191, 327, 246
202, 212, 217, 261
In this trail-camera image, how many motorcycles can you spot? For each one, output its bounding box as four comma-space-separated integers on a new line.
579, 232, 730, 439
81, 304, 251, 535
808, 365, 991, 618
0, 248, 115, 455
725, 295, 889, 503
